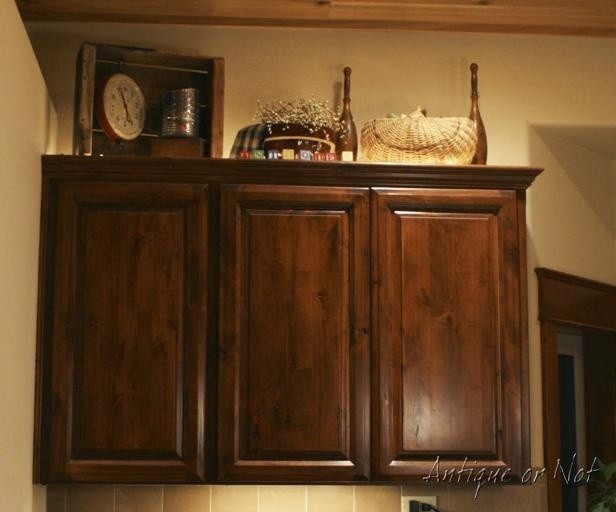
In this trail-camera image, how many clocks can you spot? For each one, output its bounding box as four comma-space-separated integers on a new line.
97, 74, 149, 141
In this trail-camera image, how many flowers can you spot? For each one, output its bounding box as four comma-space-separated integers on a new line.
250, 95, 349, 141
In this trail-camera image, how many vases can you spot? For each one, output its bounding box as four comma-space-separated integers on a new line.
260, 124, 340, 163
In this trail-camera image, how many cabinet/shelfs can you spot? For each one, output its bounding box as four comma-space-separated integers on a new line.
39, 152, 206, 485
208, 160, 546, 484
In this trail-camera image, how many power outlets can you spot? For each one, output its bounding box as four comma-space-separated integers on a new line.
399, 496, 438, 512
